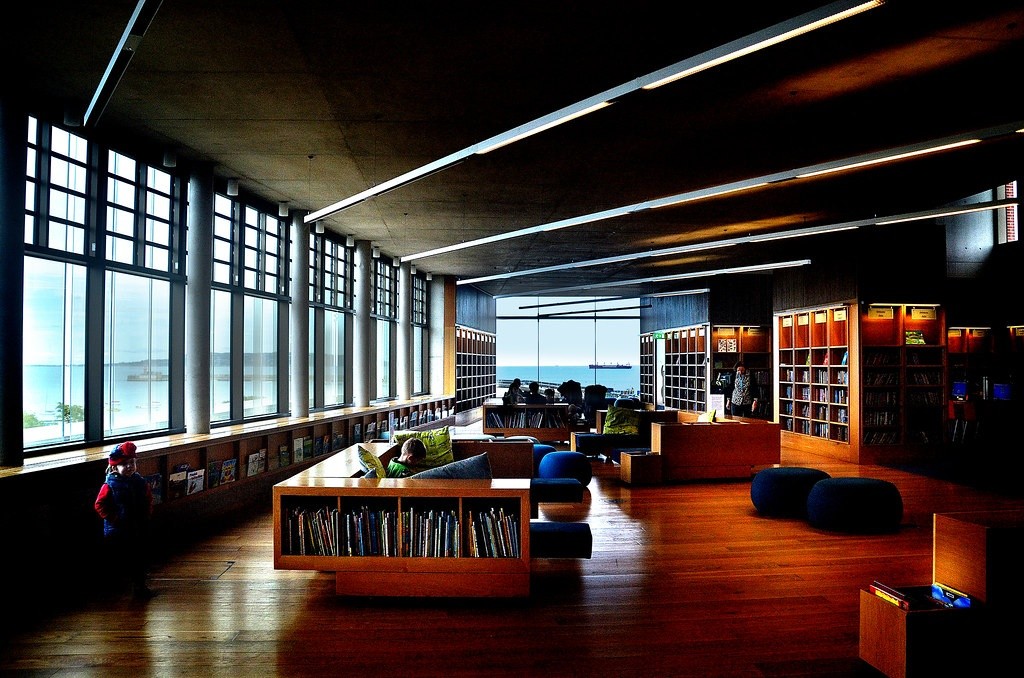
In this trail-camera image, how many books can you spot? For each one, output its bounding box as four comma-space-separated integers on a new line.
682, 363, 770, 417
267, 435, 313, 470
281, 505, 521, 559
393, 407, 454, 431
862, 351, 944, 445
871, 579, 972, 611
208, 448, 266, 489
143, 463, 205, 504
313, 419, 387, 457
786, 351, 848, 442
486, 411, 567, 429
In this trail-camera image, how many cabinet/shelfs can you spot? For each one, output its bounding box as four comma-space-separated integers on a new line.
773, 299, 946, 463
272, 327, 569, 596
639, 323, 772, 422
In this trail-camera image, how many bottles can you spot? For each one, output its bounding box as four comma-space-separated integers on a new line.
982, 376, 989, 400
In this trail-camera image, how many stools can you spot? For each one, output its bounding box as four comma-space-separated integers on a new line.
538, 452, 593, 487
533, 444, 557, 477
808, 477, 904, 535
751, 467, 830, 519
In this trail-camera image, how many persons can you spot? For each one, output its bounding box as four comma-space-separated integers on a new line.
385, 438, 427, 478
725, 361, 760, 417
503, 378, 553, 403
94, 440, 154, 601
568, 404, 579, 425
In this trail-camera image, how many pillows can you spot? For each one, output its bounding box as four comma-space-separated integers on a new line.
357, 425, 493, 480
602, 405, 640, 434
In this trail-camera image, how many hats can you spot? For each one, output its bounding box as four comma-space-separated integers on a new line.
109, 442, 136, 466
